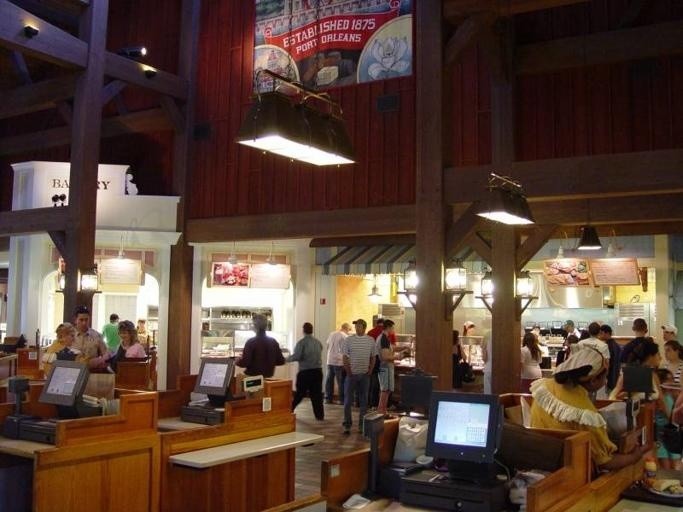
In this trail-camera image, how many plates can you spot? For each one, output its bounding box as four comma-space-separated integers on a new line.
224, 277, 236, 285
659, 381, 681, 391
647, 485, 682, 499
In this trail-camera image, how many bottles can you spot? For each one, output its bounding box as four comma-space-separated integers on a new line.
220, 310, 271, 318
644, 455, 659, 473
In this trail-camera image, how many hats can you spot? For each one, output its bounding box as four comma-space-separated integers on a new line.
353, 319, 367, 326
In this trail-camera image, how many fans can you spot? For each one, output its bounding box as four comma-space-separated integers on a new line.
217, 242, 255, 264
258, 243, 292, 268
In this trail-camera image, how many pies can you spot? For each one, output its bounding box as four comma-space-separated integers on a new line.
653, 478, 683, 494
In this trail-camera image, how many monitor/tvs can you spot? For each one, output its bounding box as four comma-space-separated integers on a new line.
38, 360, 89, 418
194, 357, 235, 405
539, 356, 551, 369
425, 390, 504, 483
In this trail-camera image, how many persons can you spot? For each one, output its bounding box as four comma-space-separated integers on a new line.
338, 318, 370, 434
452, 317, 682, 479
231, 311, 286, 378
367, 318, 398, 411
285, 320, 325, 422
36, 302, 216, 380
320, 320, 349, 405
374, 315, 404, 416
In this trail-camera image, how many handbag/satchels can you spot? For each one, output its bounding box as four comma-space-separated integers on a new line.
662, 409, 682, 453
460, 361, 476, 383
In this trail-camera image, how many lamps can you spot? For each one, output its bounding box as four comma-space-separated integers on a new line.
470, 168, 539, 229
363, 276, 385, 300
76, 264, 102, 296
53, 264, 70, 295
395, 259, 539, 321
234, 70, 359, 176
48, 190, 68, 209
19, 16, 159, 82
573, 221, 606, 253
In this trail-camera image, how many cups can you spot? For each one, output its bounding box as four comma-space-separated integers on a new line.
416, 454, 434, 470
214, 267, 223, 284
